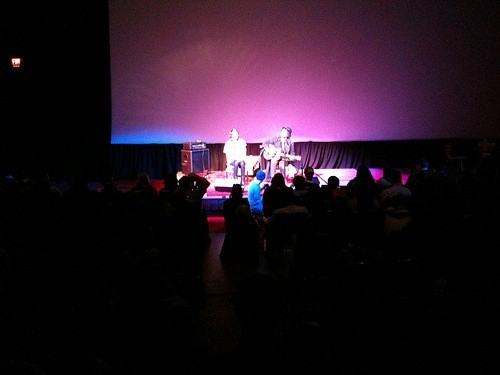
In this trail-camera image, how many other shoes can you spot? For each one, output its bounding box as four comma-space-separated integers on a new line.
241, 175, 245, 186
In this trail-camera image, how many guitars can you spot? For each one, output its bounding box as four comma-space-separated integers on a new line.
263, 143, 302, 162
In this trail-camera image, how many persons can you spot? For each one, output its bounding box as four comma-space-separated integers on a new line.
261, 125, 295, 183
222, 127, 248, 188
0, 165, 500, 375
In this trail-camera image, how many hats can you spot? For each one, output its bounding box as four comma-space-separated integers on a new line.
256, 170, 266, 181
283, 126, 292, 138
229, 184, 243, 196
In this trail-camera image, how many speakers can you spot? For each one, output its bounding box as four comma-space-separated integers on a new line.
181, 149, 211, 176
